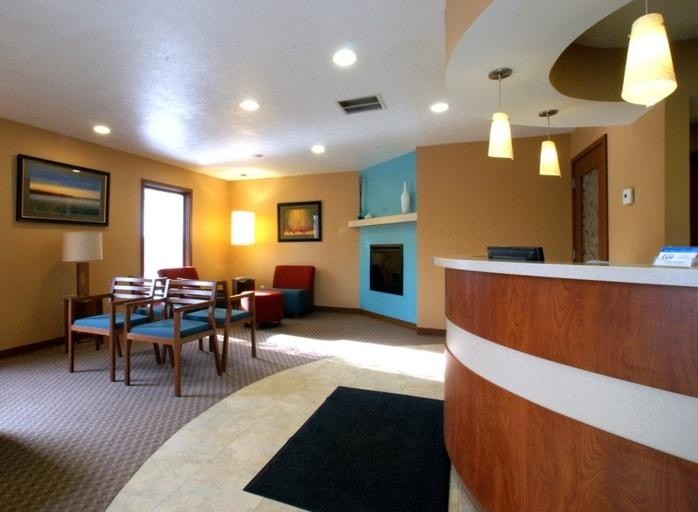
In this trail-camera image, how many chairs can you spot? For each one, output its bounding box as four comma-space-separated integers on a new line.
263, 265, 315, 318
67, 267, 257, 397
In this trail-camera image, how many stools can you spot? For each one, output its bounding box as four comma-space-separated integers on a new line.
239, 289, 283, 328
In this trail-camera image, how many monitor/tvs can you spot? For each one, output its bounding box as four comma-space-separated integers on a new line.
487, 246, 546, 263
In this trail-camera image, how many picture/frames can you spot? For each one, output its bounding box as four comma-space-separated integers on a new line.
17, 154, 110, 226
276, 200, 322, 242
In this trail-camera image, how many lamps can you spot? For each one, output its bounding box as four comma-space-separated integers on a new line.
620, 0, 678, 107
538, 110, 562, 177
488, 68, 514, 161
60, 230, 104, 296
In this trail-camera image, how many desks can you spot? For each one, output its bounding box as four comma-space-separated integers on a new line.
61, 294, 104, 354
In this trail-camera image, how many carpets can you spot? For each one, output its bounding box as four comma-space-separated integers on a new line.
243, 385, 451, 512
